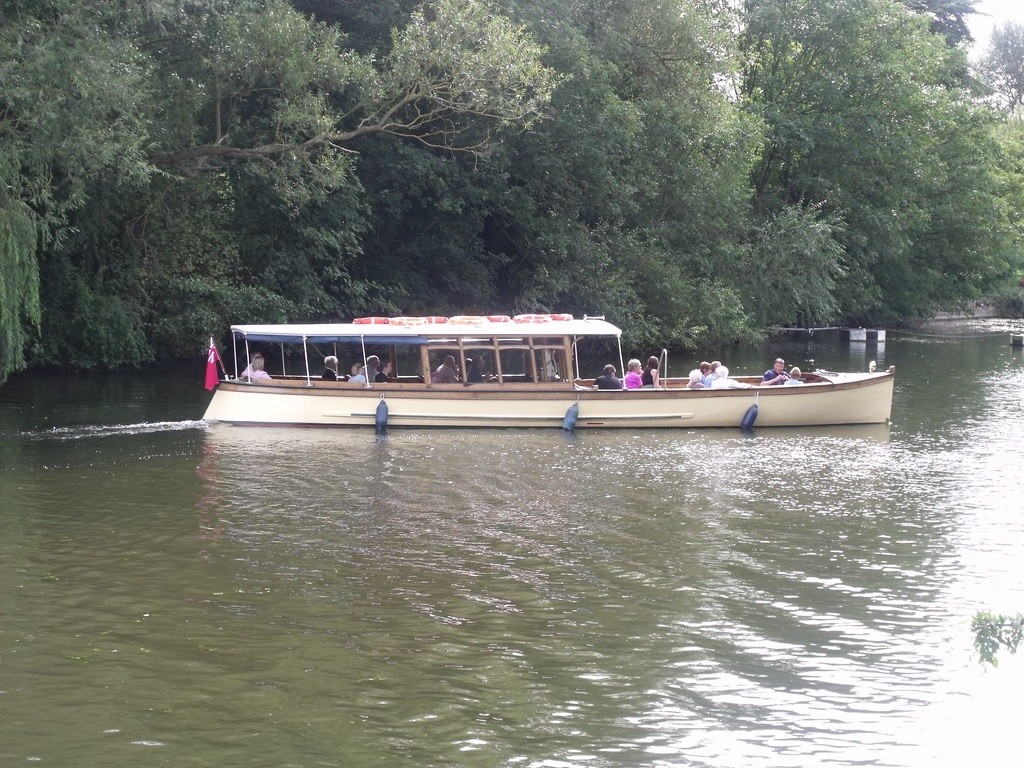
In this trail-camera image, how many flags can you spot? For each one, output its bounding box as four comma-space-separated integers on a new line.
204, 334, 220, 391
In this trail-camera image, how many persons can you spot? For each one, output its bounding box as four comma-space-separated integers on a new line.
539, 348, 560, 382
622, 356, 664, 390
348, 354, 393, 383
322, 355, 340, 381
592, 364, 621, 389
417, 352, 491, 383
241, 351, 272, 382
686, 361, 753, 390
760, 358, 804, 386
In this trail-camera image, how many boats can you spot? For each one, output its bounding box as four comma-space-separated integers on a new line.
203, 311, 895, 428
